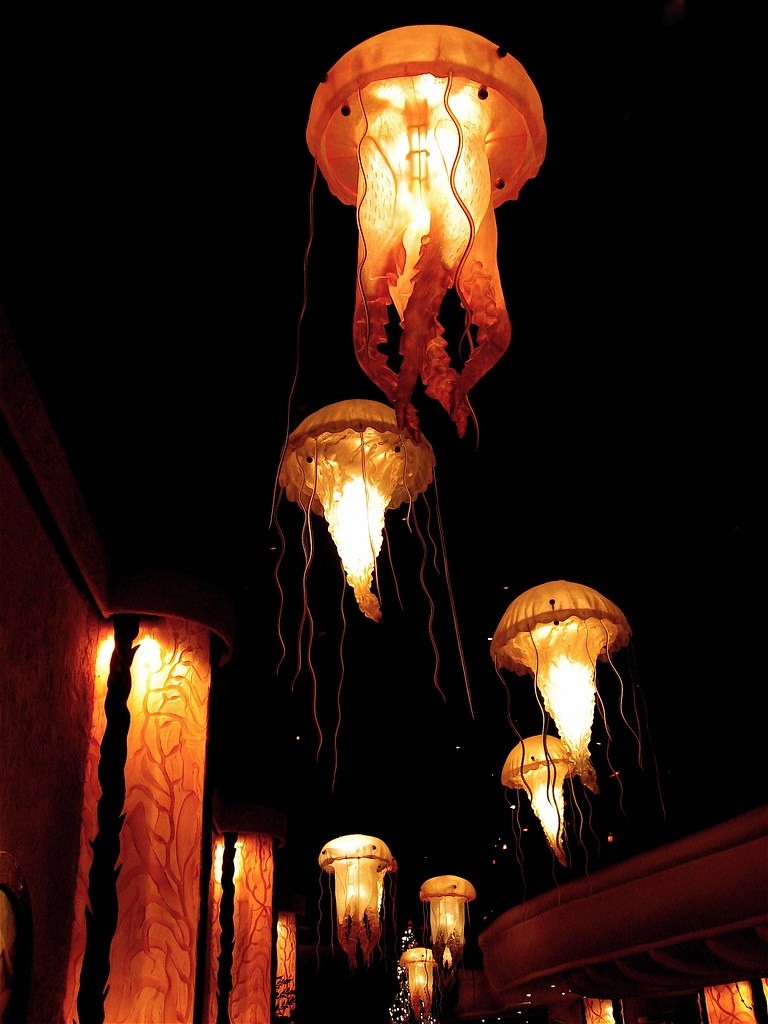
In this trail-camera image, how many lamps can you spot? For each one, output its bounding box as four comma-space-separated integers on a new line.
62, 401, 768, 1023
304, 22, 548, 447
